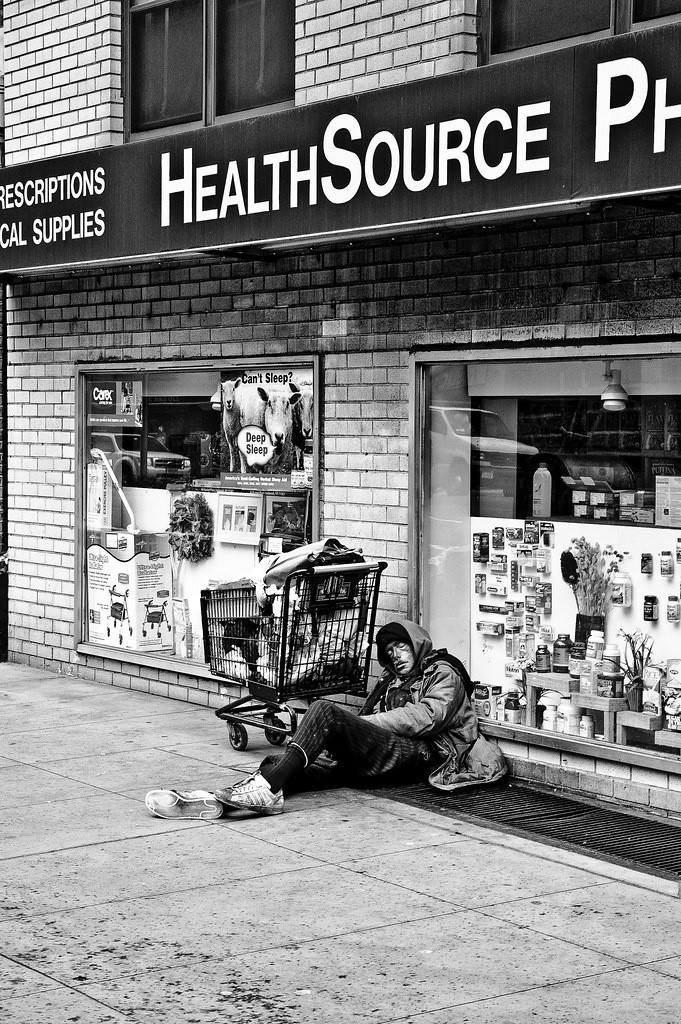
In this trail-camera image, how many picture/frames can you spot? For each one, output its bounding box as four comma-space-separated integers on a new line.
214, 492, 264, 546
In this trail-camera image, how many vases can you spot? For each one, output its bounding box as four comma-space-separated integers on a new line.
625, 684, 643, 712
575, 614, 606, 650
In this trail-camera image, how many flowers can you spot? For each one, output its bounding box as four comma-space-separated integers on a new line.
601, 628, 668, 694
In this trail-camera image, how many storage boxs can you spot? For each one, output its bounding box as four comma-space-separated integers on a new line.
87, 462, 122, 533
590, 493, 614, 506
637, 491, 656, 507
643, 667, 666, 693
470, 518, 555, 720
572, 491, 590, 505
171, 597, 191, 627
633, 510, 655, 523
573, 505, 593, 518
593, 506, 615, 520
643, 691, 669, 716
620, 492, 638, 507
175, 625, 193, 658
89, 530, 174, 652
667, 658, 681, 688
618, 506, 642, 521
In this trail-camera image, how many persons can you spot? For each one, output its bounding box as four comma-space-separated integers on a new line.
145, 620, 508, 820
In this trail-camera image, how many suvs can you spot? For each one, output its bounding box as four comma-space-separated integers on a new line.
431, 405, 541, 497
91, 433, 192, 487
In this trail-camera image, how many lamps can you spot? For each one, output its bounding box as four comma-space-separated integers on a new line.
601, 360, 629, 411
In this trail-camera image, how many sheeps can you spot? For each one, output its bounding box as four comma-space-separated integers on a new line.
211, 373, 312, 474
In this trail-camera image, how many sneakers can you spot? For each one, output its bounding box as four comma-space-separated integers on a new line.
145, 785, 225, 821
208, 772, 286, 816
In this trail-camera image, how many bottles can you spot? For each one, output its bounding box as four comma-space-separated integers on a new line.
640, 537, 681, 624
504, 629, 621, 741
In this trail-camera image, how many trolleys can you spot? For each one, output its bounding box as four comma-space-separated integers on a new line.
200, 561, 388, 751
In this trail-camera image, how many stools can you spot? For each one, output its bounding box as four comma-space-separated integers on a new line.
655, 730, 681, 749
526, 672, 580, 728
616, 711, 663, 745
572, 692, 630, 743
